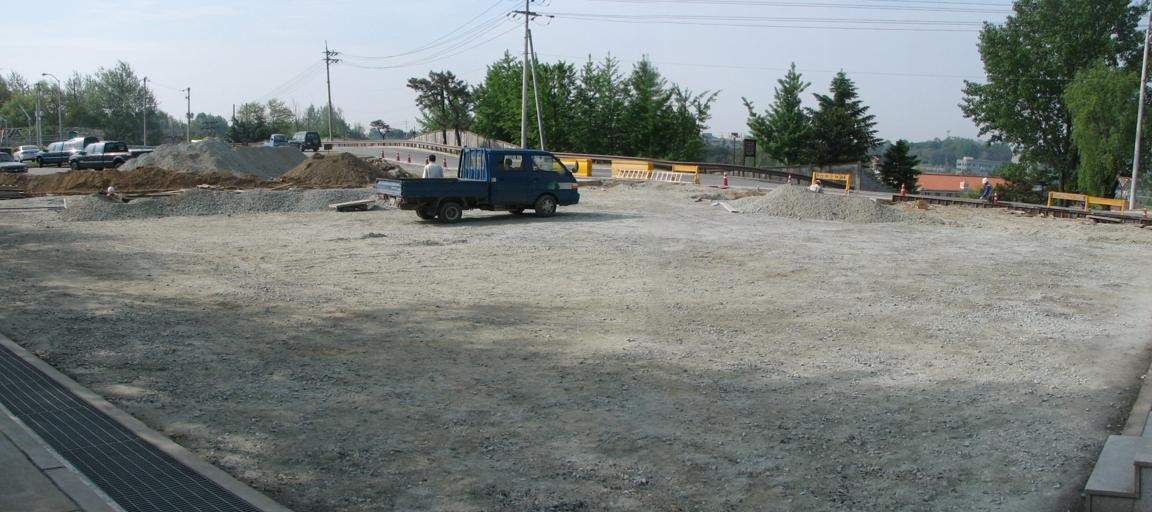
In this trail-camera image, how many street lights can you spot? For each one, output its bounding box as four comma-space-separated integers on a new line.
1128, 0, 1152, 210
142, 76, 152, 147
42, 72, 64, 141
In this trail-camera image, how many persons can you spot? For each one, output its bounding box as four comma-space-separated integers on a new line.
13, 148, 21, 162
422, 155, 444, 178
980, 177, 993, 199
809, 180, 822, 193
106, 186, 120, 201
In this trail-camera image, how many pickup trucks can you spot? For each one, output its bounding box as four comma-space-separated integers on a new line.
0, 136, 154, 172
375, 147, 581, 223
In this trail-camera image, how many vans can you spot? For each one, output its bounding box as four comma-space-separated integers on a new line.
269, 131, 322, 151
